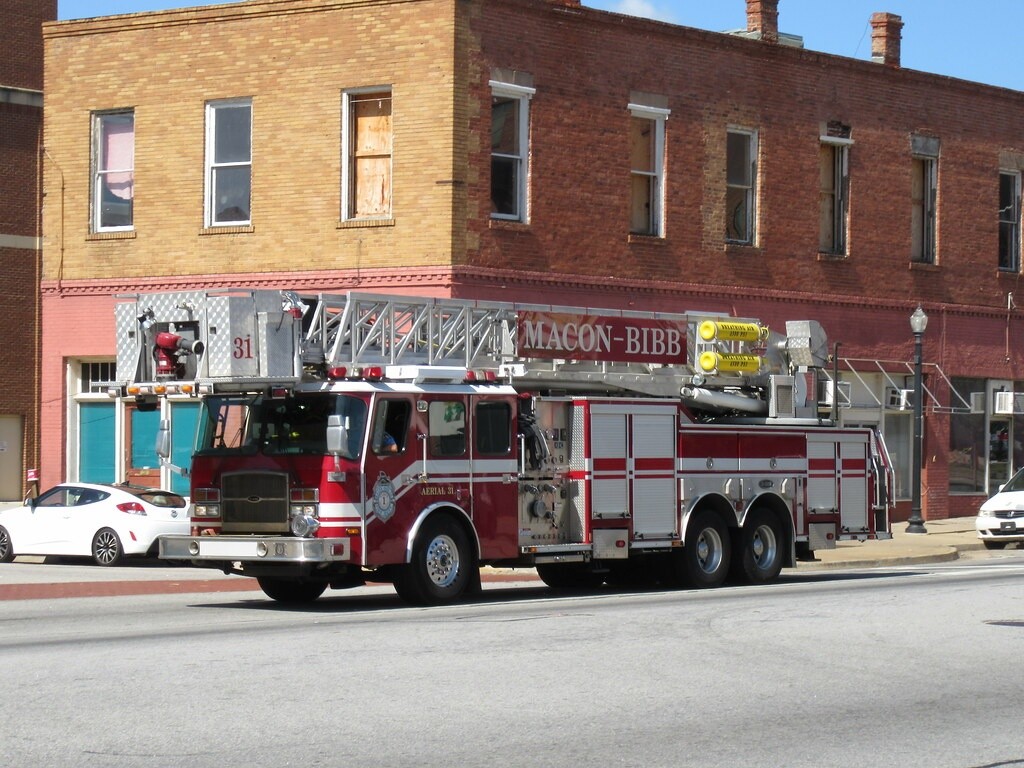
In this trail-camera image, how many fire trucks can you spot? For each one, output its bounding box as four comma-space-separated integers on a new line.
97, 287, 898, 610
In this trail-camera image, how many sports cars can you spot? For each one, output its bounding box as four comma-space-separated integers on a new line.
0, 478, 193, 565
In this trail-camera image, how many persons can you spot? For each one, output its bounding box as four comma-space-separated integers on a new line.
348, 411, 397, 457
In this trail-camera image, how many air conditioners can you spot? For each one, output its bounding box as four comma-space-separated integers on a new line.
818, 380, 850, 407
970, 392, 985, 413
901, 390, 917, 411
995, 393, 1024, 414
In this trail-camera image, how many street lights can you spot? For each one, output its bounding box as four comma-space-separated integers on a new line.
903, 306, 928, 534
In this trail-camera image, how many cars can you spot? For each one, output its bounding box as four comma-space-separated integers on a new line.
975, 467, 1024, 550
